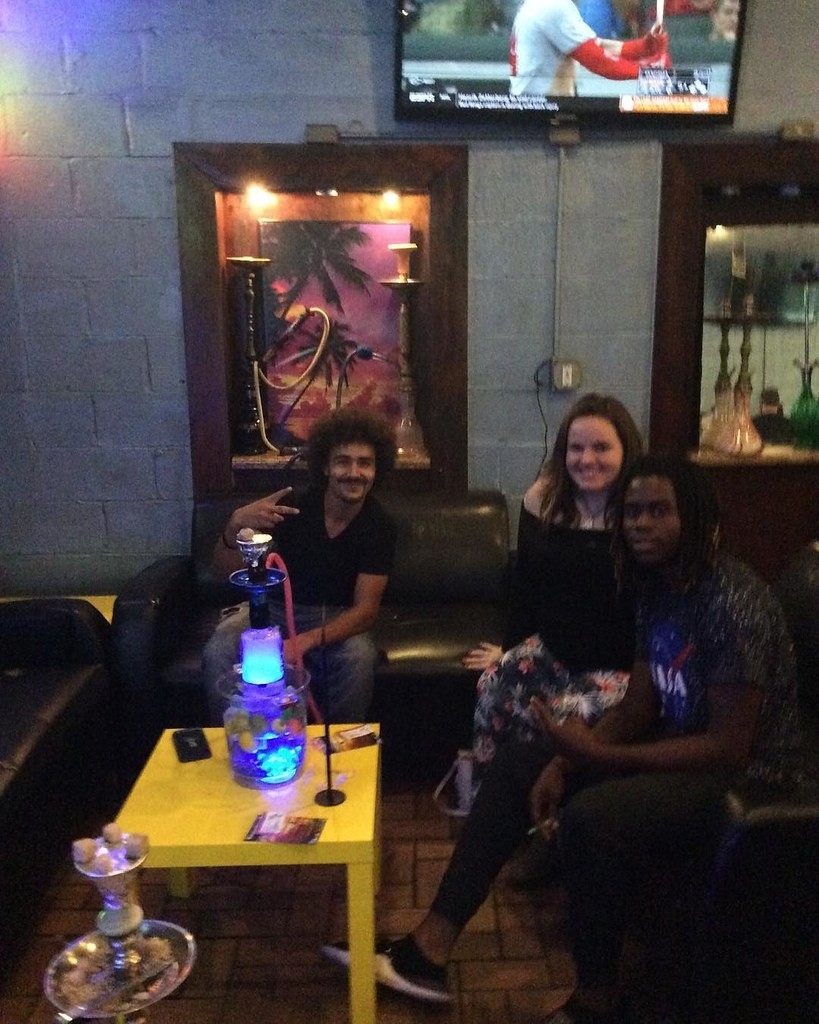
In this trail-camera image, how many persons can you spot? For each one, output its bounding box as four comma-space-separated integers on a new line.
507, 0, 741, 99
200, 404, 402, 724
460, 392, 643, 783
324, 446, 805, 1024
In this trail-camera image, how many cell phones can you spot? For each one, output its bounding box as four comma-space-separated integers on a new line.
173, 727, 213, 762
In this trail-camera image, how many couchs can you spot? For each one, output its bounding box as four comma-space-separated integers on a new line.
621, 539, 819, 1024
0, 599, 112, 988
111, 487, 509, 761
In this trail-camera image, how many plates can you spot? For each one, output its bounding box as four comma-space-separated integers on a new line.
42, 920, 196, 1019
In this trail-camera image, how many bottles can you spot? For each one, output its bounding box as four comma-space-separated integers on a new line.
793, 370, 815, 447
809, 390, 819, 450
698, 374, 734, 449
715, 375, 762, 457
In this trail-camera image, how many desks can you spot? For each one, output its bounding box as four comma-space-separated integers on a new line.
693, 445, 819, 567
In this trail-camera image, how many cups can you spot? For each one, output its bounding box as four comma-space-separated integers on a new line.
215, 663, 311, 790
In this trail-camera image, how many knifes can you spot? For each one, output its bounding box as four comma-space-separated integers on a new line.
52, 957, 177, 1024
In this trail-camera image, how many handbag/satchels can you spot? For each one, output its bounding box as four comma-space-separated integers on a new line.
433, 749, 483, 818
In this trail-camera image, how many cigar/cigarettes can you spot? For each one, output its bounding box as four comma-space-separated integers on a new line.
525, 819, 551, 837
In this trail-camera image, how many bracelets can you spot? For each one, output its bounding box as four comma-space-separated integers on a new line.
221, 531, 238, 552
320, 625, 326, 647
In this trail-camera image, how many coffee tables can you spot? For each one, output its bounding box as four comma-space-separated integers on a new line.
111, 722, 383, 1024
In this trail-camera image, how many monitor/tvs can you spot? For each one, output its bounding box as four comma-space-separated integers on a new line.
393, 0, 747, 127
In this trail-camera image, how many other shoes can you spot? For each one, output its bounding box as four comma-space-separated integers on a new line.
534, 990, 637, 1024
322, 944, 456, 1004
